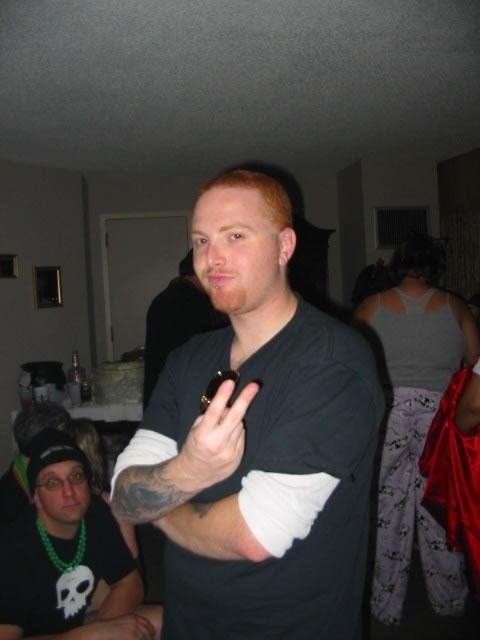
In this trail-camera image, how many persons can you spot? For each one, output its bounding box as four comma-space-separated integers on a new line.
139, 245, 230, 608
106, 168, 391, 639
1, 399, 166, 640
350, 234, 479, 632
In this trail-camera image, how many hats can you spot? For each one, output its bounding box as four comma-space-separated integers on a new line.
27, 427, 92, 497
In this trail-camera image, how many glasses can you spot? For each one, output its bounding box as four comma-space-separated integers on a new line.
35, 472, 86, 492
198, 369, 241, 415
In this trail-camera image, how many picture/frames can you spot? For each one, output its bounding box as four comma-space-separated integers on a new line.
0, 253, 22, 280
33, 265, 63, 308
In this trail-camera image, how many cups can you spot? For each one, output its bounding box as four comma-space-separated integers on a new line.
17, 384, 58, 409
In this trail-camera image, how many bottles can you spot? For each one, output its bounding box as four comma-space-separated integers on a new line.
69, 351, 92, 404
